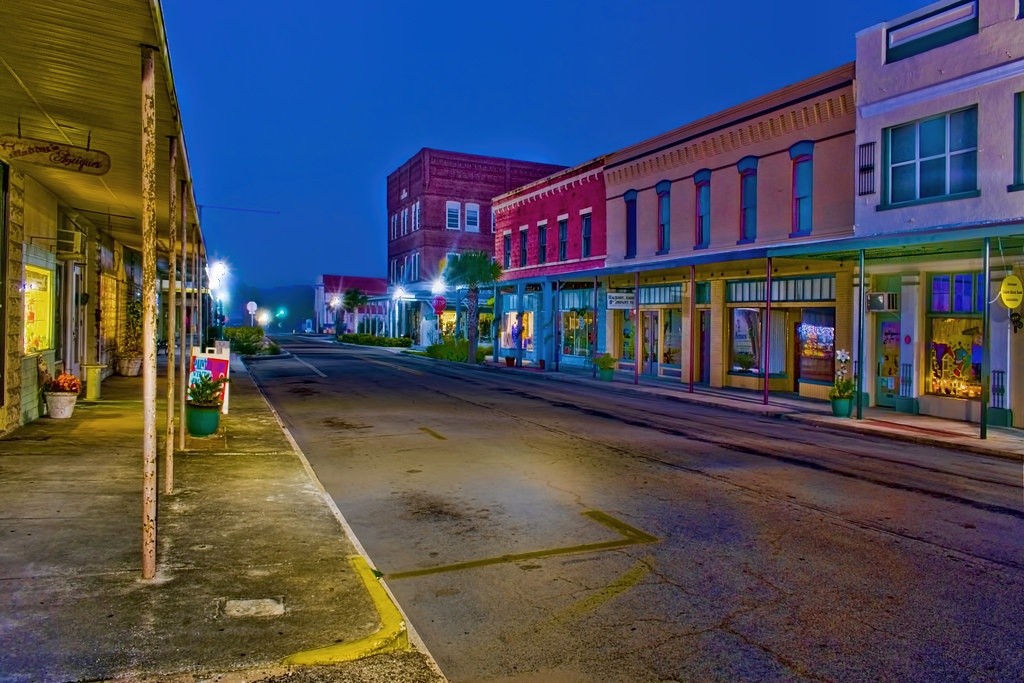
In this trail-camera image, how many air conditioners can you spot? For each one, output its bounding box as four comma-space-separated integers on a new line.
866, 292, 900, 312
57, 229, 88, 255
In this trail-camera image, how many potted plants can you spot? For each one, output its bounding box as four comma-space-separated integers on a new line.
734, 352, 756, 373
115, 299, 143, 376
185, 373, 233, 438
592, 353, 618, 382
43, 374, 81, 419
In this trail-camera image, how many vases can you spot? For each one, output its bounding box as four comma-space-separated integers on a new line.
540, 360, 545, 368
831, 397, 856, 418
505, 357, 515, 367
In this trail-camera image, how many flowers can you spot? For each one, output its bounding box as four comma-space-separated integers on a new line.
829, 349, 856, 397
